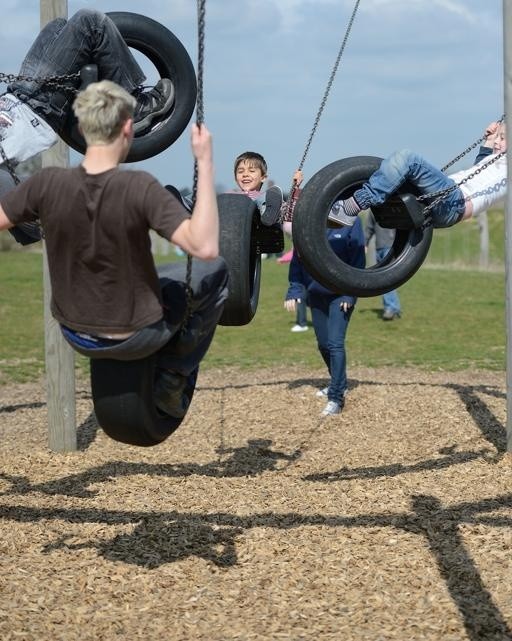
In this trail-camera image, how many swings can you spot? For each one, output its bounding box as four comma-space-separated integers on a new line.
1, 0, 510, 448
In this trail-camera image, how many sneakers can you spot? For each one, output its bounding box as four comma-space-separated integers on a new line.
379, 308, 403, 323
257, 184, 285, 225
324, 199, 359, 228
149, 373, 191, 419
316, 384, 348, 420
288, 323, 309, 334
127, 75, 175, 135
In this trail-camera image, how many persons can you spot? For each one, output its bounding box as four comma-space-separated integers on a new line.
283, 215, 366, 418
0, 81, 232, 420
277, 222, 309, 333
164, 150, 304, 256
364, 209, 402, 320
325, 121, 507, 232
0, 7, 175, 169
